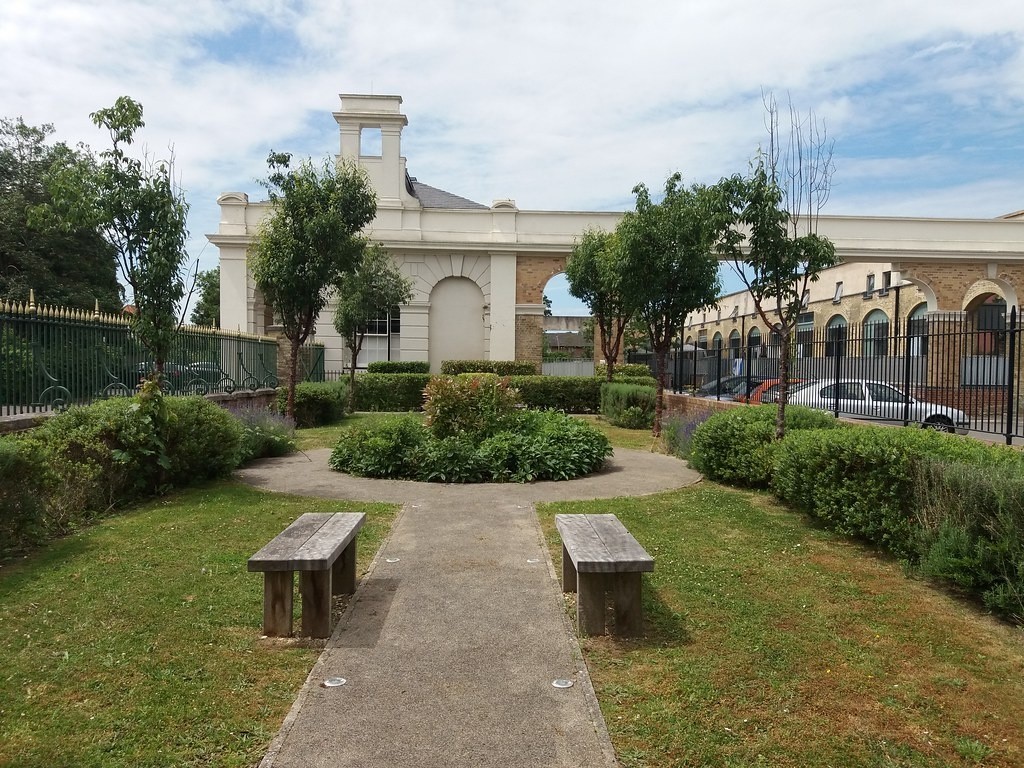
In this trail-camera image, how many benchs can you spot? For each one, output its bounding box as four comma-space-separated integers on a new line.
247, 512, 367, 639
554, 513, 654, 637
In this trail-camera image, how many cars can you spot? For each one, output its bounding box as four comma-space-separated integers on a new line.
697, 376, 778, 401
775, 379, 970, 435
741, 379, 809, 404
104, 362, 221, 394
